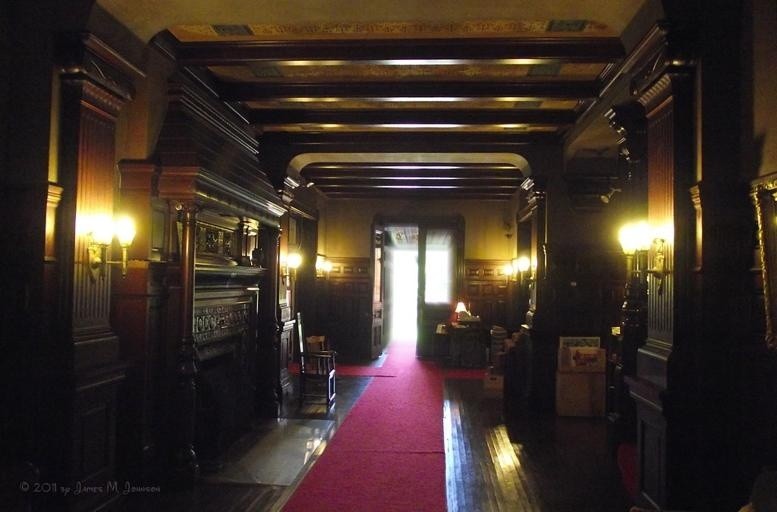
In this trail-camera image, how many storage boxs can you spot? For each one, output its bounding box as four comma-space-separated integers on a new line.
551, 334, 608, 424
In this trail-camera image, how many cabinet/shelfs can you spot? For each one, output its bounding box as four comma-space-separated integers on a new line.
116, 160, 287, 477
434, 320, 487, 367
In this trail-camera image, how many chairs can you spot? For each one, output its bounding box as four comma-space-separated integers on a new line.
295, 311, 339, 412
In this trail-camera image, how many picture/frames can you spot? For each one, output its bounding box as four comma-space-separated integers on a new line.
747, 172, 777, 352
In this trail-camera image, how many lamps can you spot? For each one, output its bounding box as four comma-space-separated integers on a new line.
616, 222, 670, 291
76, 211, 137, 283
454, 301, 469, 328
501, 256, 539, 288
